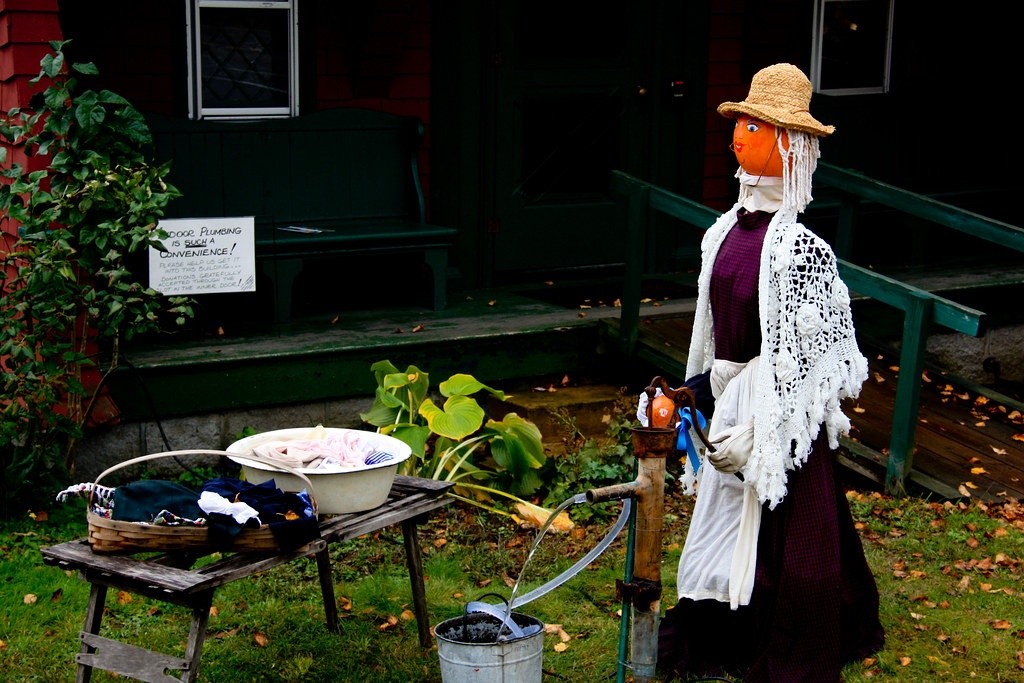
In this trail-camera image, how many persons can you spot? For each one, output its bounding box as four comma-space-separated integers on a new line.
637, 59, 888, 683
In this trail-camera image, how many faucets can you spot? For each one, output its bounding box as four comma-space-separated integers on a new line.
586, 423, 665, 682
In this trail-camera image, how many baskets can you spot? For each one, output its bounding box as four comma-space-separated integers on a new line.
87, 449, 319, 553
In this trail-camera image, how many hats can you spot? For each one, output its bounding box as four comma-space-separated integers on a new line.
716, 62, 834, 137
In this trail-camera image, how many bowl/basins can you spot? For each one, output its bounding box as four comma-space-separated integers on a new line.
227, 427, 412, 514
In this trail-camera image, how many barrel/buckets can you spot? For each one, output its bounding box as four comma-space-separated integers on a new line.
434, 593, 545, 683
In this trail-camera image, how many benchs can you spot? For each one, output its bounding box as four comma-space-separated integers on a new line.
106, 107, 460, 345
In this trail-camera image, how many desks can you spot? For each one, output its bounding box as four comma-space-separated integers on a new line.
38, 474, 458, 683
